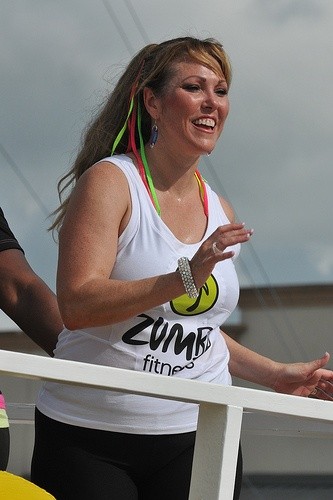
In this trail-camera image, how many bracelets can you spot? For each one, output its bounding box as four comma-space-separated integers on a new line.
177, 255, 199, 300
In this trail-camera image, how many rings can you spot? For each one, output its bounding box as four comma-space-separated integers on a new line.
209, 240, 222, 255
310, 389, 318, 397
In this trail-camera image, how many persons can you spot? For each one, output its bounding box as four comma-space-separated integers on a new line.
0, 203, 65, 472
45, 36, 333, 499
